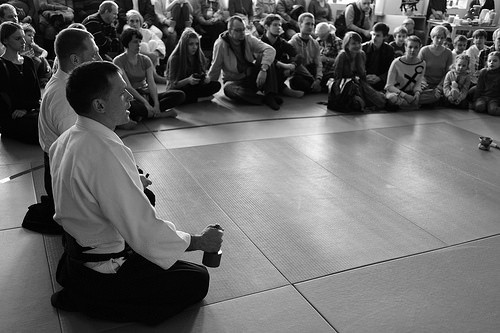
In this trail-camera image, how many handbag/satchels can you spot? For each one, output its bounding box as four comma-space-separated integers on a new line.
288, 4, 305, 20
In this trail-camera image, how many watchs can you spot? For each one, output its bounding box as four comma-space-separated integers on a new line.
260, 68, 268, 73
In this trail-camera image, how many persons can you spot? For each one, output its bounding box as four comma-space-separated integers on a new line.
361, 22, 396, 94
0, 21, 42, 146
384, 35, 440, 111
48, 60, 224, 327
113, 28, 185, 122
417, 25, 453, 105
326, 31, 400, 114
287, 12, 333, 94
21, 29, 156, 236
0, 0, 344, 89
432, 54, 471, 111
473, 51, 500, 116
333, 0, 373, 44
166, 28, 221, 106
394, 0, 500, 109
207, 16, 284, 111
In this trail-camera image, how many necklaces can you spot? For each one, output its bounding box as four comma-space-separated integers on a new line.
4, 53, 23, 74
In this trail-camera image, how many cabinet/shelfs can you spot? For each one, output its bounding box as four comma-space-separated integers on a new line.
381, 15, 426, 45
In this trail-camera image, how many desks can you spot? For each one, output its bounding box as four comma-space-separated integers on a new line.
424, 19, 500, 49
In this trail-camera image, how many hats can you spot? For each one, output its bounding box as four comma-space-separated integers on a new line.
314, 22, 336, 34
